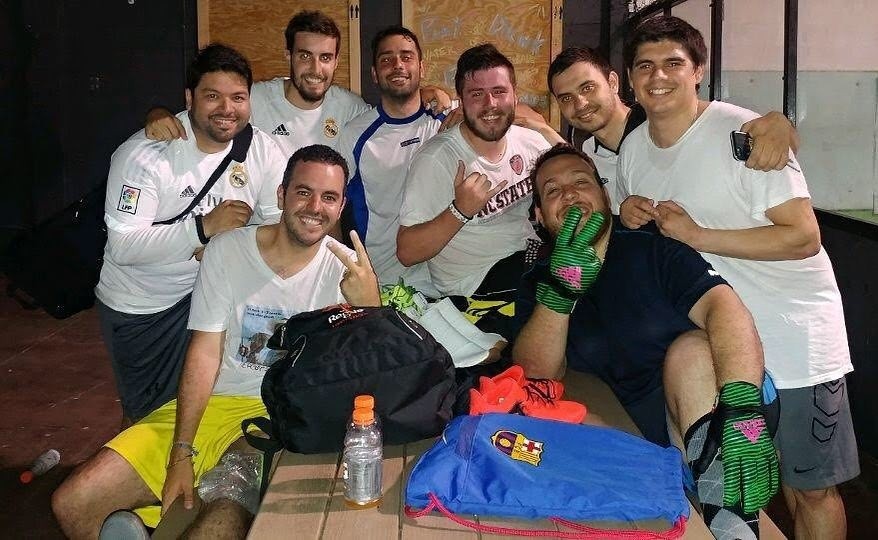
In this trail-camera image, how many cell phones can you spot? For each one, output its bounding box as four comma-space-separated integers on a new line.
730, 130, 753, 160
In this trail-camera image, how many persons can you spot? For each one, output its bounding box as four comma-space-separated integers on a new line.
476, 142, 781, 540
95, 10, 800, 428
54, 144, 380, 540
612, 15, 862, 540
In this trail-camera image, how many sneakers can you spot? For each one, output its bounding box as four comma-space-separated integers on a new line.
479, 366, 565, 400
470, 378, 587, 424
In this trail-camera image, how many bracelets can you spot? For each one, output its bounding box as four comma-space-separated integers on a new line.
546, 274, 581, 305
448, 199, 474, 224
166, 442, 198, 469
194, 215, 208, 245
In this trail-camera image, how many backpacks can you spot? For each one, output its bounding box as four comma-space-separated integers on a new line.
241, 305, 503, 455
405, 413, 690, 540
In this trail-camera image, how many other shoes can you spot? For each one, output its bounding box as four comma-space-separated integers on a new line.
98, 509, 151, 540
381, 276, 502, 350
381, 292, 490, 369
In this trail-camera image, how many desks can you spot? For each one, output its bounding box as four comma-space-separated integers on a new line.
242, 368, 718, 540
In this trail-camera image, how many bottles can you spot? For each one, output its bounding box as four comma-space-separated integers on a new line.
342, 395, 384, 509
20, 448, 60, 483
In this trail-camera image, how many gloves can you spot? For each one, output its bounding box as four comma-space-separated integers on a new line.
695, 381, 778, 515
535, 207, 606, 316
